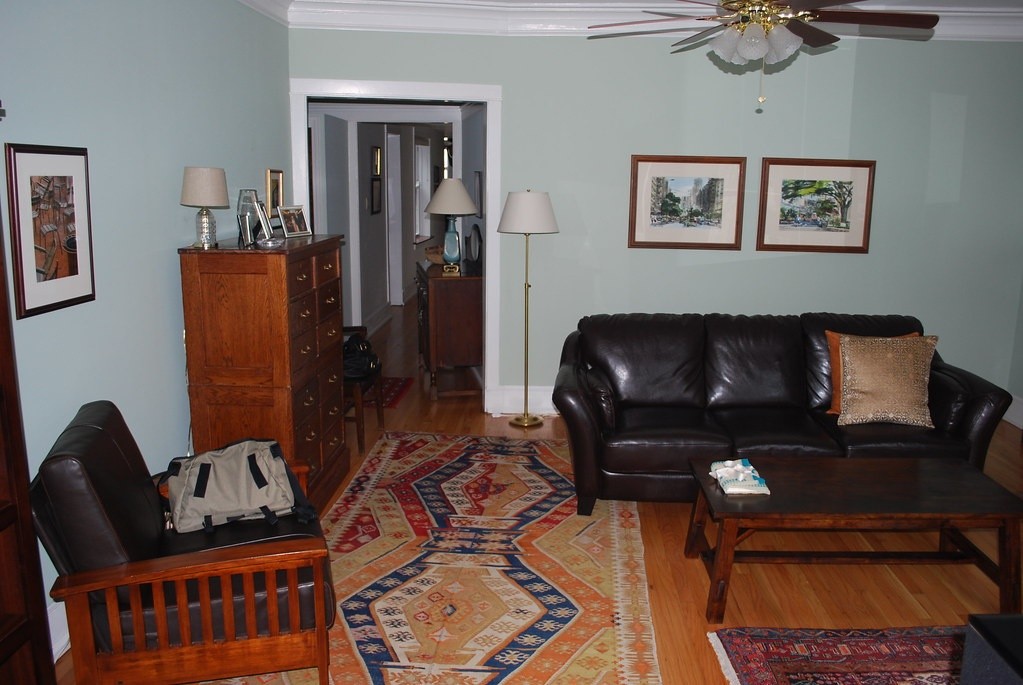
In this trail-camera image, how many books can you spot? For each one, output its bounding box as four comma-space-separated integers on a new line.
711, 458, 770, 495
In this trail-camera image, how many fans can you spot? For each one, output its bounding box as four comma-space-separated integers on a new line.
587, 0, 938, 66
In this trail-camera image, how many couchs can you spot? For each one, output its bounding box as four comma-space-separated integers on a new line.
552, 313, 1013, 516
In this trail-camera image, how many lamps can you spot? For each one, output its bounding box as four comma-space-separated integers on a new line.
424, 178, 479, 278
708, 0, 803, 103
181, 166, 230, 249
497, 189, 559, 428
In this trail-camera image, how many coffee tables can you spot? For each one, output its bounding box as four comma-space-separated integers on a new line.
684, 457, 1023, 624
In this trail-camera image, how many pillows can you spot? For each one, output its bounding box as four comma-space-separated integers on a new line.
825, 329, 919, 414
837, 333, 939, 428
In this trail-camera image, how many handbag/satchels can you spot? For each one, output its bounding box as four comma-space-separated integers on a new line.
166, 440, 296, 533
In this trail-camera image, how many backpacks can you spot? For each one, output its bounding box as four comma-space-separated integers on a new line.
341, 332, 382, 378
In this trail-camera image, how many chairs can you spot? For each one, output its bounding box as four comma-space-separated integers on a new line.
342, 326, 385, 455
28, 401, 335, 685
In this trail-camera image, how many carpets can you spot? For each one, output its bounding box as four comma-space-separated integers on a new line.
196, 433, 662, 685
705, 626, 966, 685
361, 378, 417, 410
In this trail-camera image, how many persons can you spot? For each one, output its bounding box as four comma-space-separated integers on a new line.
283, 209, 303, 232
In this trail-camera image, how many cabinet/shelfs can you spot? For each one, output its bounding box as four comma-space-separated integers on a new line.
413, 260, 485, 400
177, 234, 350, 520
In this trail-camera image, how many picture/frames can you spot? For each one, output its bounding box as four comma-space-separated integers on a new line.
755, 156, 876, 254
629, 154, 747, 251
265, 168, 284, 219
371, 146, 382, 214
3, 142, 96, 320
277, 204, 312, 238
